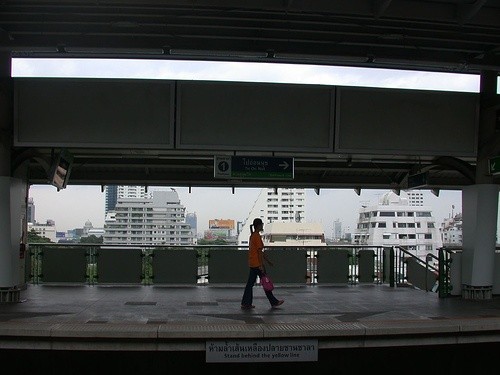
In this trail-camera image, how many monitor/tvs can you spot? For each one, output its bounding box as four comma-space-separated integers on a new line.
48, 154, 70, 189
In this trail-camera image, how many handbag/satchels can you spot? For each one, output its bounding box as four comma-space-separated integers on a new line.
260, 273, 273, 291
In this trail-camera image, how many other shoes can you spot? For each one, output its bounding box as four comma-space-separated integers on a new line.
271, 299, 285, 306
240, 304, 256, 310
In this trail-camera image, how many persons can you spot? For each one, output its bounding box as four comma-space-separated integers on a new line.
241, 219, 285, 307
